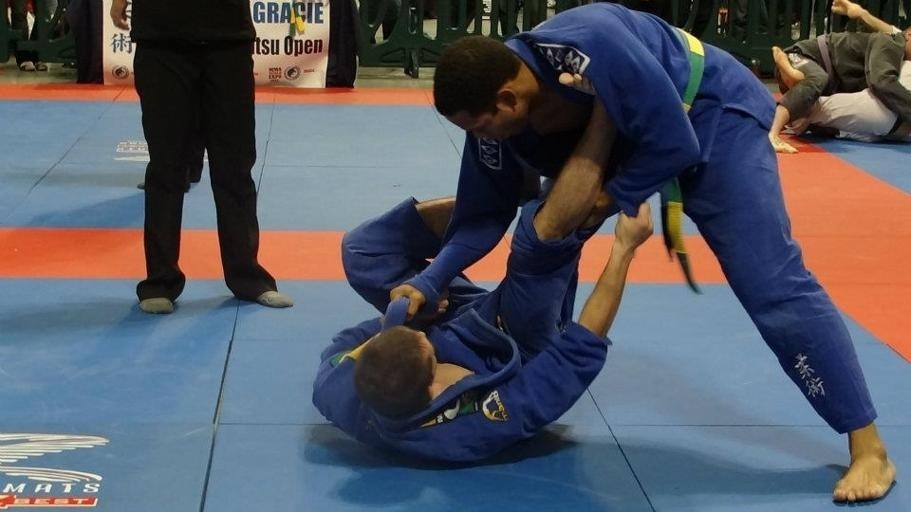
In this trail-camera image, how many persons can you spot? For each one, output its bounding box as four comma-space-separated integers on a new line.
1, 0, 103, 84
326, 1, 486, 91
619, 0, 911, 155
311, 96, 653, 466
390, 4, 897, 503
489, 0, 585, 42
108, 1, 294, 314
